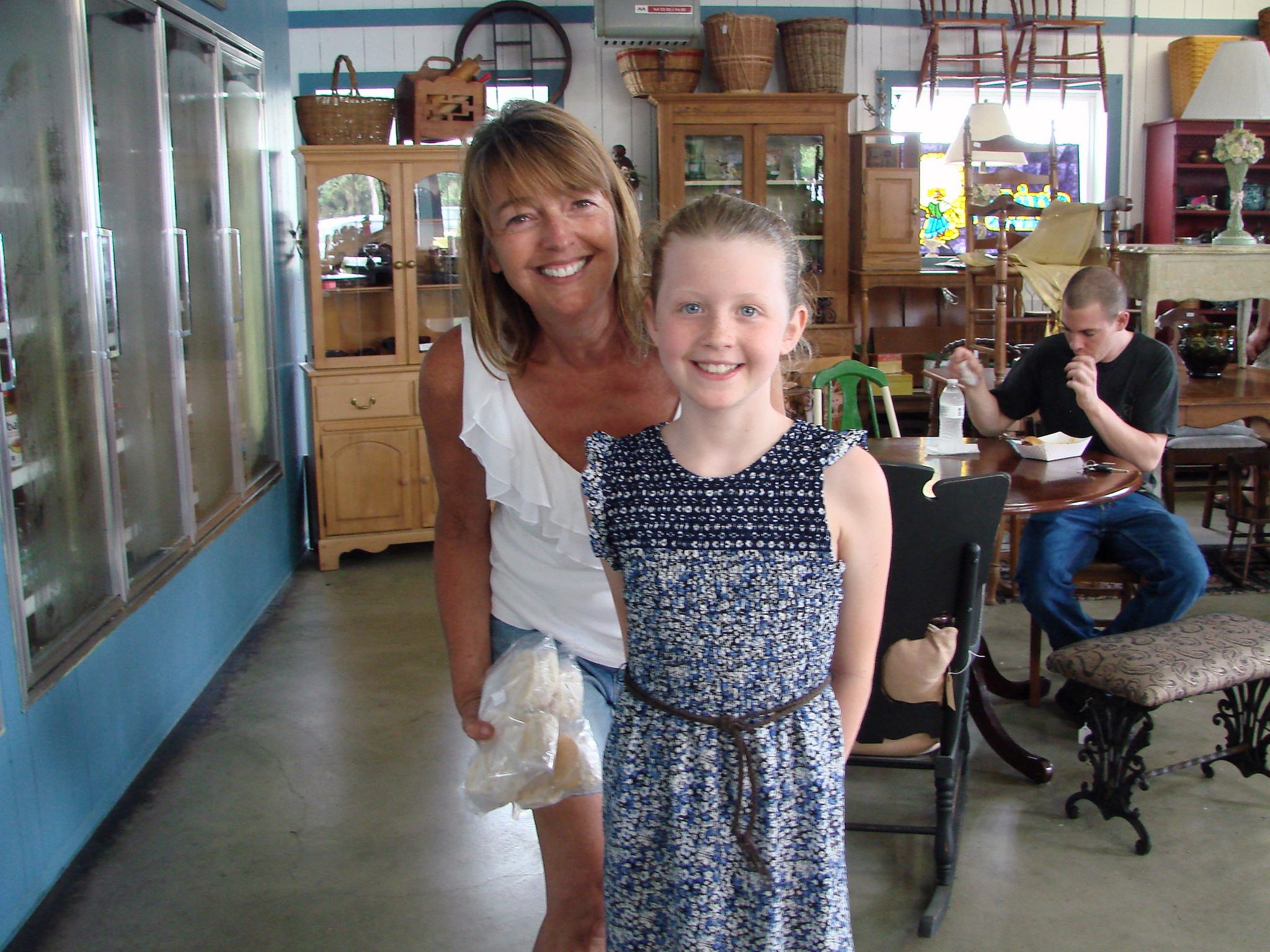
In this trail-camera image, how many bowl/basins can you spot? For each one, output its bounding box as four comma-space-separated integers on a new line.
1176, 237, 1201, 244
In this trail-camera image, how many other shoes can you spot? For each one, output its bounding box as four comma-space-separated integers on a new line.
1054, 680, 1096, 712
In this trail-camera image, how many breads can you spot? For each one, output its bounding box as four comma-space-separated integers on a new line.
1022, 436, 1043, 445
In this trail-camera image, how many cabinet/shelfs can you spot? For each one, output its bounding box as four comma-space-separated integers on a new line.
296, 144, 467, 570
645, 92, 857, 357
847, 129, 924, 273
1143, 120, 1270, 344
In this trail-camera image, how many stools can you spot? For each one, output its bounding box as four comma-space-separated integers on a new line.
1043, 612, 1270, 855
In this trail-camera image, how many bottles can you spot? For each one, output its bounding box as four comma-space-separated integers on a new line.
1178, 322, 1234, 378
939, 378, 965, 453
1191, 149, 1211, 164
1243, 183, 1265, 211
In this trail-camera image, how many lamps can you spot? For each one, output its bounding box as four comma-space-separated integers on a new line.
1181, 41, 1270, 244
943, 98, 1028, 253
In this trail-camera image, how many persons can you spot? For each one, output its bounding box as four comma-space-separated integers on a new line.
417, 100, 785, 952
947, 267, 1208, 713
579, 189, 893, 951
1215, 299, 1270, 510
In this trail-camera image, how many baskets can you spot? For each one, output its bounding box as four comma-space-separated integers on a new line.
1167, 36, 1243, 114
776, 18, 848, 92
702, 15, 776, 91
615, 48, 704, 98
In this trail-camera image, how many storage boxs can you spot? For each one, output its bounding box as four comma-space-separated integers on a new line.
864, 325, 989, 397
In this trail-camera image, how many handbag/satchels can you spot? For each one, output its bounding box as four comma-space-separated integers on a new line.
293, 55, 395, 144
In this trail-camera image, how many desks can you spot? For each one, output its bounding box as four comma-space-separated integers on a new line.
855, 393, 928, 436
848, 268, 1024, 391
862, 438, 1142, 784
922, 358, 1270, 548
1098, 242, 1270, 368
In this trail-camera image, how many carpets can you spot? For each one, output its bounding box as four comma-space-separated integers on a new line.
984, 544, 1270, 606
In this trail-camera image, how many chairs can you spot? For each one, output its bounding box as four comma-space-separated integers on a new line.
928, 337, 1150, 707
1155, 306, 1270, 581
964, 115, 1132, 381
812, 360, 903, 438
915, 0, 1111, 112
846, 462, 1013, 936
